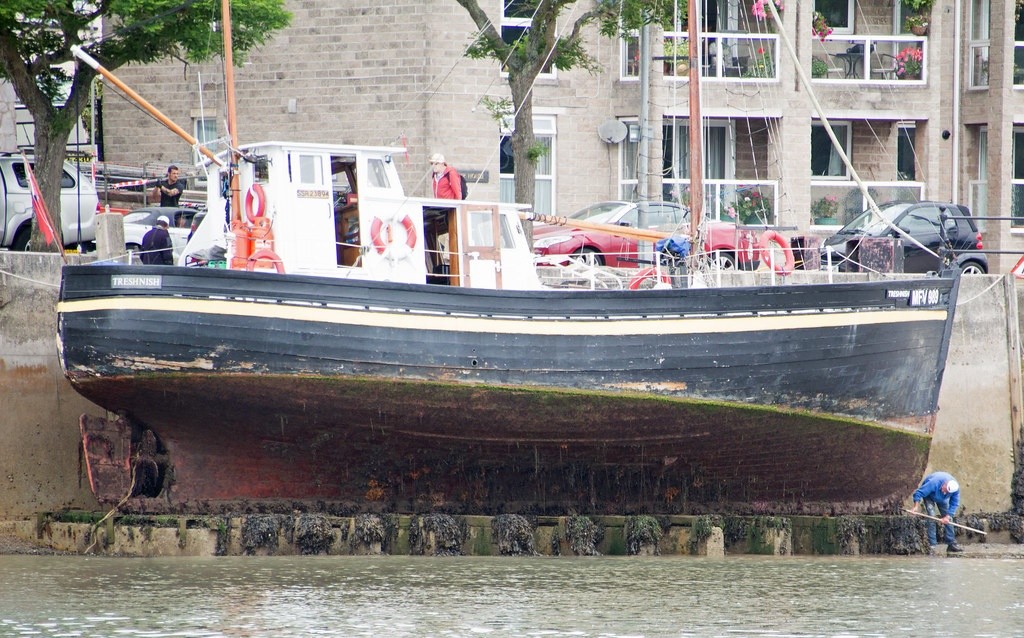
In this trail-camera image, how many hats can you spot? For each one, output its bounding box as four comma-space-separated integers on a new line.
946, 480, 959, 494
429, 153, 445, 163
156, 215, 170, 229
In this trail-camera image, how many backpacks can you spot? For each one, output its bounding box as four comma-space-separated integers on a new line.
432, 171, 468, 200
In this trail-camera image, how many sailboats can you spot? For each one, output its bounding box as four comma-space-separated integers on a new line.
52, 0, 965, 515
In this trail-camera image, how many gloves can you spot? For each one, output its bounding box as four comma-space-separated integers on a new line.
153, 179, 162, 188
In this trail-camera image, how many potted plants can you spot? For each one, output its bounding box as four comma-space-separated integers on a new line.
843, 188, 879, 225
812, 55, 828, 78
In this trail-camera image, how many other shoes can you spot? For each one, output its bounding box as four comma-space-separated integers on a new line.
946, 543, 963, 552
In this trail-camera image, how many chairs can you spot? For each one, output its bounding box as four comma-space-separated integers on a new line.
826, 54, 846, 79
709, 42, 743, 77
872, 54, 899, 79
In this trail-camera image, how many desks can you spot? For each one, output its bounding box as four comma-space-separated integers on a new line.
828, 53, 863, 79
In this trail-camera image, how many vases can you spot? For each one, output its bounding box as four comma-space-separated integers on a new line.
813, 218, 838, 225
766, 11, 773, 18
744, 216, 764, 224
911, 27, 926, 35
904, 75, 917, 79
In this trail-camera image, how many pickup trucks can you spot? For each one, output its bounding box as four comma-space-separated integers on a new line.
0, 156, 101, 252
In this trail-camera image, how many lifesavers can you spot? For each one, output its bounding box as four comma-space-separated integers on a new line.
371, 209, 417, 257
243, 183, 264, 224
759, 229, 795, 276
629, 269, 671, 291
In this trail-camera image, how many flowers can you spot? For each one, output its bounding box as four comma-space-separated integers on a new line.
669, 187, 736, 218
752, 0, 784, 18
896, 47, 923, 77
730, 182, 772, 221
904, 15, 928, 32
810, 194, 848, 219
812, 11, 833, 42
742, 48, 774, 78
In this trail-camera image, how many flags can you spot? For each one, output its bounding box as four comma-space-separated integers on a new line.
21, 150, 57, 247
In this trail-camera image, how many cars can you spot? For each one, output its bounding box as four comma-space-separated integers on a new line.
532, 201, 761, 277
820, 202, 989, 275
121, 208, 199, 256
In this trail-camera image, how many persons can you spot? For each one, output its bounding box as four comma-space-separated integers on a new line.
176, 213, 188, 229
430, 153, 462, 199
140, 215, 173, 266
909, 471, 964, 554
153, 166, 183, 208
